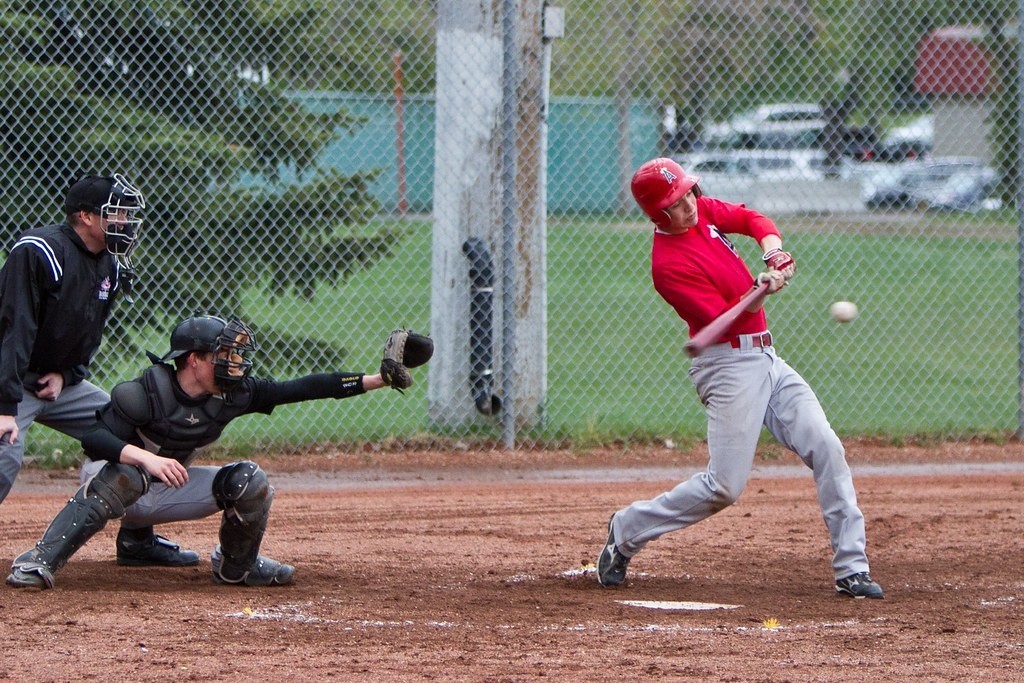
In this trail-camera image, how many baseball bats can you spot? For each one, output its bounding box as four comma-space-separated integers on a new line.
683, 280, 769, 358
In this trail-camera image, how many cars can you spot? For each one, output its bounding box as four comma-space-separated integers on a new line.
667, 103, 1006, 212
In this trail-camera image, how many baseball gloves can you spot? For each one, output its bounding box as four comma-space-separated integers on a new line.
380, 328, 434, 389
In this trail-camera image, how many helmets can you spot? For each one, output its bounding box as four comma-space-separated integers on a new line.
162, 315, 228, 361
631, 158, 702, 229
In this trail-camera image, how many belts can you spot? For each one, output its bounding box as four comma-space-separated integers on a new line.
731, 333, 771, 348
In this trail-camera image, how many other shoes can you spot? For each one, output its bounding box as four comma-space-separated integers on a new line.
6, 565, 46, 591
116, 527, 200, 566
211, 545, 295, 587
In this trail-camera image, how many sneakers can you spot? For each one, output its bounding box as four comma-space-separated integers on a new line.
835, 572, 883, 599
597, 513, 630, 589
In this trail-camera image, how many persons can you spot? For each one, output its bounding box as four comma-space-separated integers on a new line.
595, 154, 885, 599
4, 315, 434, 590
1, 170, 202, 566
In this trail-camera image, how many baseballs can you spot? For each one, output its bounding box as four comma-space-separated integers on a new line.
829, 300, 858, 322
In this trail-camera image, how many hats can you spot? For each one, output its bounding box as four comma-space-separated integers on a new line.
62, 175, 146, 219
